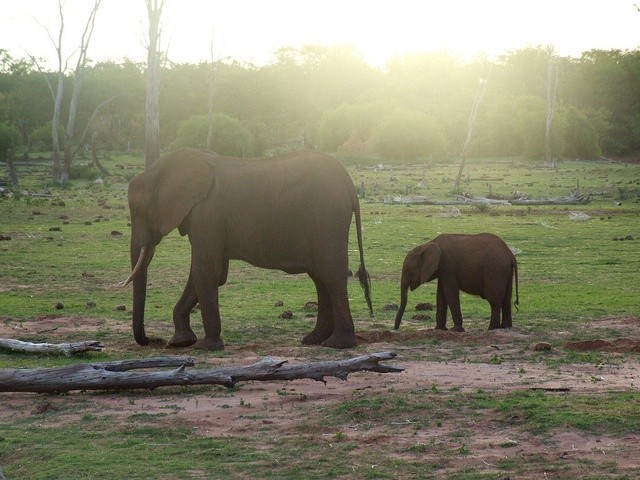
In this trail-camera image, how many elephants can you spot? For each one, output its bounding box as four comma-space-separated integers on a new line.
393, 232, 519, 333
117, 147, 374, 351
